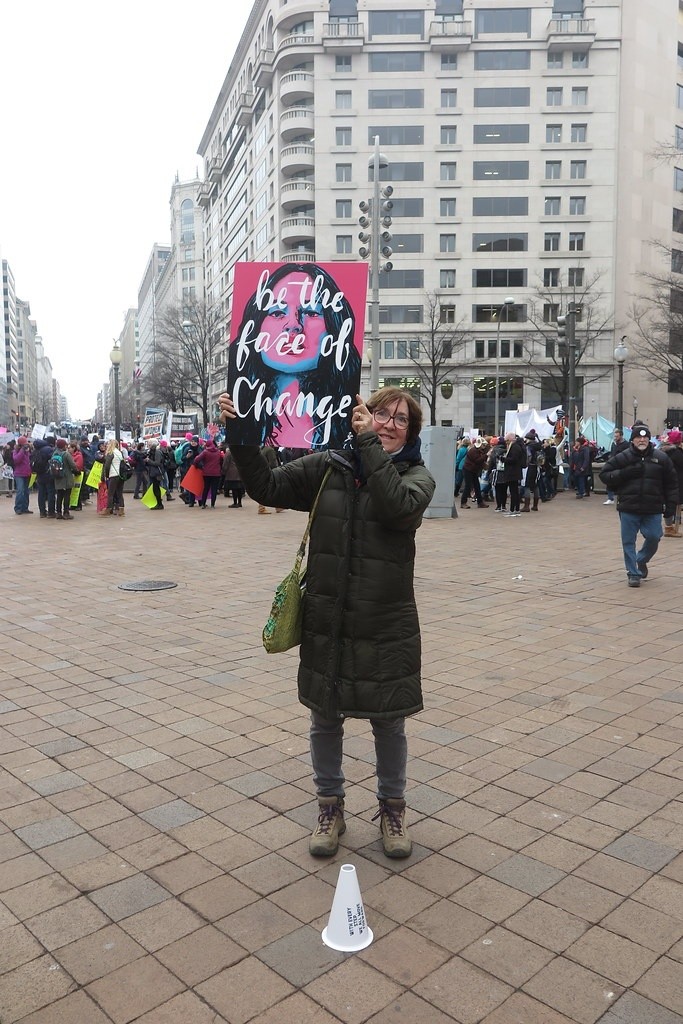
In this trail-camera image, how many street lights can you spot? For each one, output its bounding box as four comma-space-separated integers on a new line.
494, 297, 515, 437
182, 320, 213, 426
109, 345, 123, 451
613, 340, 628, 436
357, 134, 394, 399
633, 399, 638, 424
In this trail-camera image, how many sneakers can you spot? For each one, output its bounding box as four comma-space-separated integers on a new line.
627, 573, 640, 587
309, 796, 347, 857
637, 562, 648, 578
372, 798, 412, 858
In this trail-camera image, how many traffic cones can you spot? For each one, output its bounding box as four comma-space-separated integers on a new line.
321, 863, 375, 955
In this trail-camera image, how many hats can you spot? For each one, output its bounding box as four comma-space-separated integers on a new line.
630, 420, 651, 439
525, 429, 535, 440
185, 433, 213, 449
160, 441, 167, 446
18, 436, 28, 445
667, 433, 682, 444
504, 432, 516, 442
57, 439, 67, 447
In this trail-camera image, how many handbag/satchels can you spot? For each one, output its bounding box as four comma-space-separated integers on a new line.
262, 571, 301, 653
497, 460, 505, 471
455, 464, 459, 472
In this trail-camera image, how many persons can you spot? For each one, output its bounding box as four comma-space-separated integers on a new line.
455, 409, 599, 516
218, 386, 435, 861
603, 420, 683, 537
599, 423, 679, 586
0, 420, 285, 519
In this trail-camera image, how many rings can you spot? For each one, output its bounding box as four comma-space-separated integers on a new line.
358, 413, 361, 419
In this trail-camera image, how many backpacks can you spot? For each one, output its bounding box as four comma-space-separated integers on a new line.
174, 442, 190, 465
29, 448, 48, 476
112, 452, 133, 480
50, 452, 66, 478
536, 451, 546, 465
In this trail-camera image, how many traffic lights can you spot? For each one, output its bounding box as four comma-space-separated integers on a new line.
15, 412, 18, 417
558, 315, 569, 347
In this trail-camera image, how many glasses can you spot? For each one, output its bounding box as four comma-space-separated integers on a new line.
374, 409, 409, 430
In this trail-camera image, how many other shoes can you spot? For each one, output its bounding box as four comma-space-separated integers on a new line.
6, 490, 283, 520
453, 491, 683, 537
